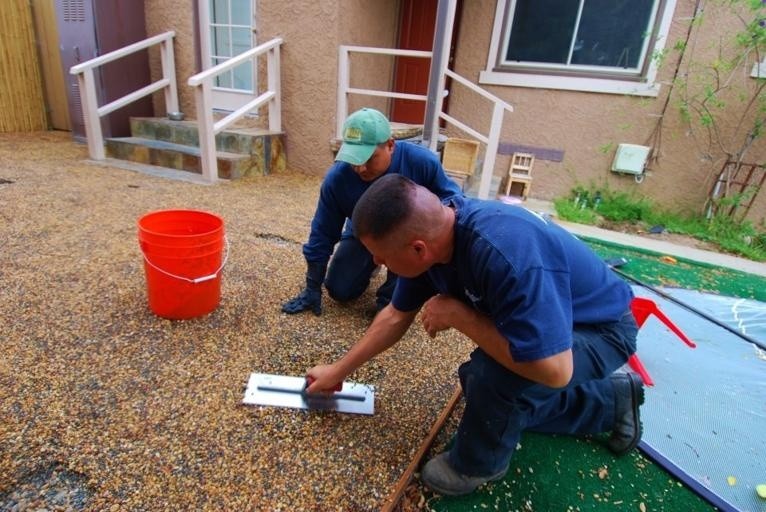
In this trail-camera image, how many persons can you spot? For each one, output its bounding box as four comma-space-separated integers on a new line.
282, 105, 467, 320
302, 171, 660, 500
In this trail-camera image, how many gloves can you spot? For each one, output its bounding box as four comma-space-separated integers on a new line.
376, 272, 397, 304
281, 259, 328, 316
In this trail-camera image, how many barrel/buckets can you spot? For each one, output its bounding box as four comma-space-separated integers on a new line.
138, 209, 230, 319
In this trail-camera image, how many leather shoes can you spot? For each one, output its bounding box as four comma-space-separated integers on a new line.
422, 451, 510, 496
608, 372, 645, 457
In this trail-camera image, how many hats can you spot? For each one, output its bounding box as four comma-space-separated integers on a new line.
334, 108, 392, 165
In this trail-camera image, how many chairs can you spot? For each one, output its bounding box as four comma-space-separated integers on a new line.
502, 151, 535, 201
